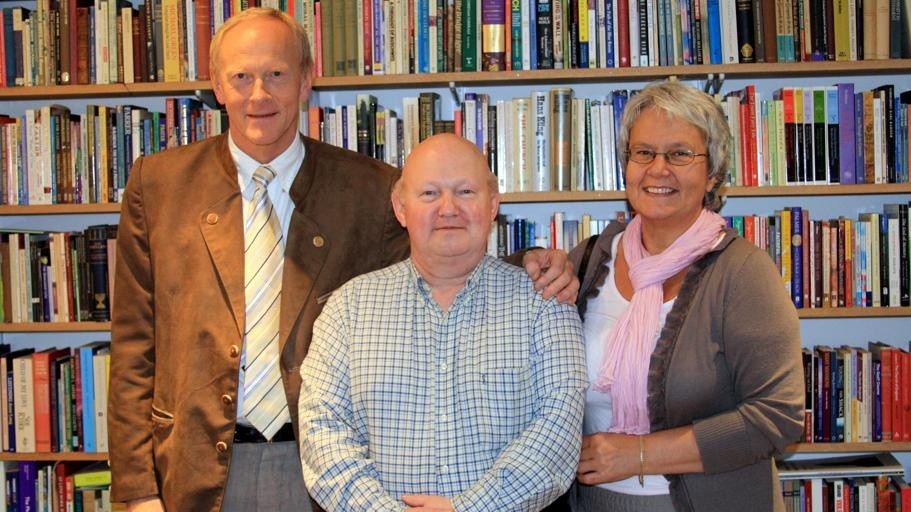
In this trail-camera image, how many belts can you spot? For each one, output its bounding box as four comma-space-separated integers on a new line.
233, 422, 295, 443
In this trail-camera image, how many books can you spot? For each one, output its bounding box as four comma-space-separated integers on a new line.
0, 1, 911, 512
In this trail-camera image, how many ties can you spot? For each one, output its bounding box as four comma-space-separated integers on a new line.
243, 165, 289, 444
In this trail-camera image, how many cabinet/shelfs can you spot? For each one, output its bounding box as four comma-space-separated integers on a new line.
1, 0, 911, 512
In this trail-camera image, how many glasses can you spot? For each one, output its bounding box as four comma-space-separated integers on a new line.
627, 147, 708, 166
